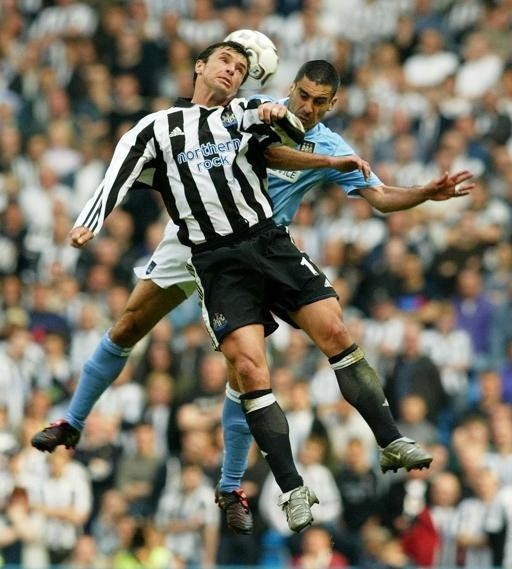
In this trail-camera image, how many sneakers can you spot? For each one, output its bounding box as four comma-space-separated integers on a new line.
32, 419, 81, 453
216, 485, 253, 536
378, 436, 433, 474
279, 485, 319, 532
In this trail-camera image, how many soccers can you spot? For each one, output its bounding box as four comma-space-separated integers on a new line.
224, 29, 278, 89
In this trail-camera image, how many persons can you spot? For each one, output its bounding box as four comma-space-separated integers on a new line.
0, 0, 510, 568
